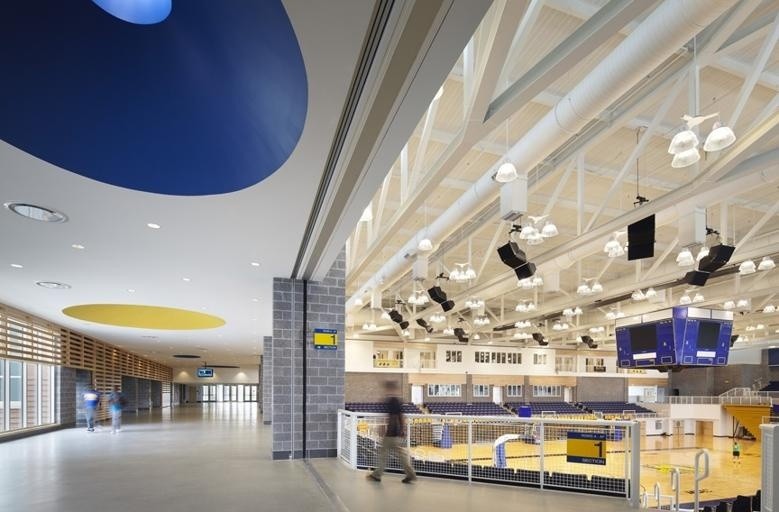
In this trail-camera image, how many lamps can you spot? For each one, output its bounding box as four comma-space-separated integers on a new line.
350, 161, 779, 351
666, 35, 736, 171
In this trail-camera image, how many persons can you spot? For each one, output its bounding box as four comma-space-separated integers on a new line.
732, 442, 740, 461
77, 384, 124, 431
367, 381, 420, 483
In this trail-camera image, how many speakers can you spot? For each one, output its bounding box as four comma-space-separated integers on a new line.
729, 336, 739, 347
454, 328, 468, 342
533, 334, 548, 345
685, 246, 735, 286
388, 311, 409, 329
416, 318, 433, 332
427, 287, 455, 311
497, 240, 536, 280
582, 336, 597, 348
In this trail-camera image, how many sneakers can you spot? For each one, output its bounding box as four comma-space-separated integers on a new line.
369, 474, 411, 483
